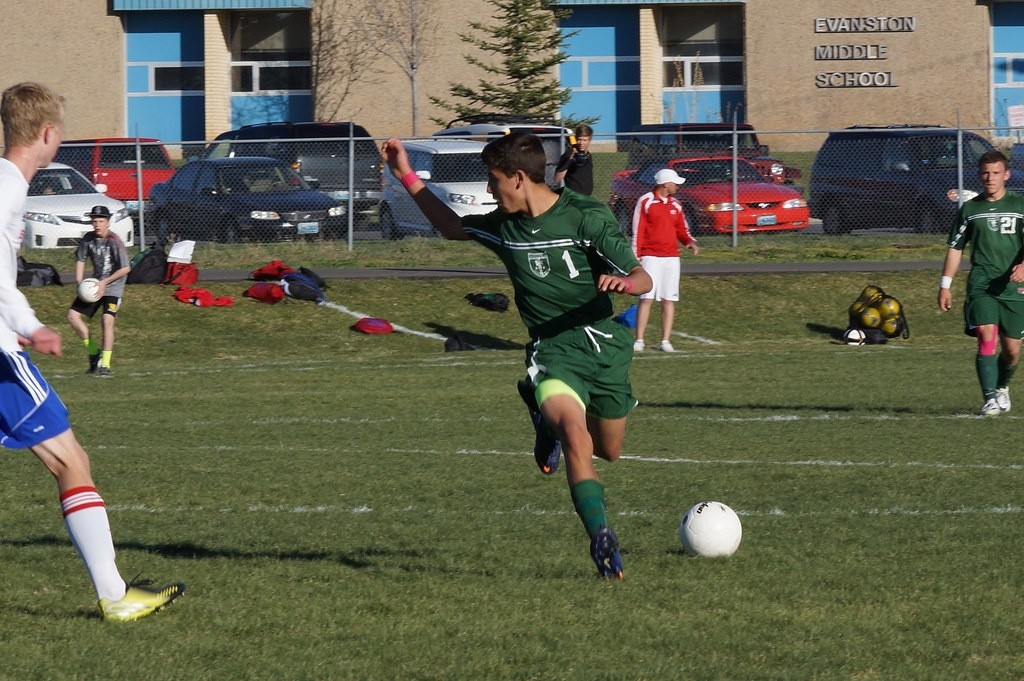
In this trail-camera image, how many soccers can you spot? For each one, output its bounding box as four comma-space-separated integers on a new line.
879, 318, 898, 334
862, 307, 882, 328
77, 278, 104, 302
878, 297, 900, 320
850, 302, 868, 319
863, 286, 883, 306
680, 501, 743, 556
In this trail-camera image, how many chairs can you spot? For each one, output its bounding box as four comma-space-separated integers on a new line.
252, 180, 272, 191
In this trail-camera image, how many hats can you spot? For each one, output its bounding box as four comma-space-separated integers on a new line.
654, 169, 686, 184
85, 206, 114, 217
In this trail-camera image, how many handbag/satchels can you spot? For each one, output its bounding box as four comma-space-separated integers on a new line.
281, 266, 329, 304
252, 260, 294, 279
247, 283, 285, 304
16, 256, 64, 286
126, 244, 199, 286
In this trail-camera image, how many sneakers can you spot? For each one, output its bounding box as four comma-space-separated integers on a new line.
94, 365, 112, 379
982, 398, 1001, 416
85, 348, 103, 376
996, 385, 1011, 412
658, 340, 674, 352
634, 339, 645, 351
96, 571, 185, 623
590, 528, 624, 591
531, 410, 561, 475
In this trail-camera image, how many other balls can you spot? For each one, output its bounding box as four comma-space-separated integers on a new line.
843, 328, 867, 347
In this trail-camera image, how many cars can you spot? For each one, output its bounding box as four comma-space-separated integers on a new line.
606, 152, 810, 237
20, 159, 136, 251
144, 155, 348, 254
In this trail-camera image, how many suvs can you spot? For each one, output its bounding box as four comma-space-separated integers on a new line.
185, 120, 383, 232
51, 135, 177, 220
429, 112, 579, 194
806, 122, 1024, 237
626, 122, 805, 200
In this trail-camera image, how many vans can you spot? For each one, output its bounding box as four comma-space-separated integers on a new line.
376, 136, 502, 243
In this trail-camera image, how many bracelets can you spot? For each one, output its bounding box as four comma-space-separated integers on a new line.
939, 275, 952, 289
622, 277, 633, 294
401, 170, 419, 187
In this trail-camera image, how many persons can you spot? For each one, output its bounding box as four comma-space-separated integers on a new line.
0, 82, 190, 626
65, 206, 132, 377
553, 125, 593, 198
630, 169, 698, 352
380, 132, 654, 580
938, 152, 1024, 415
